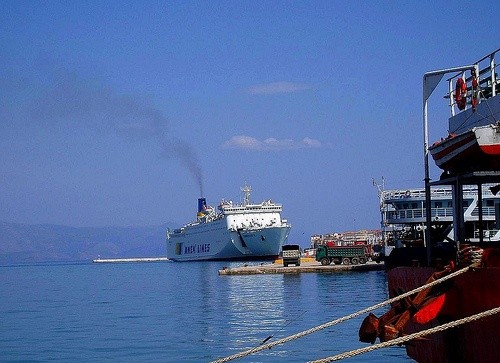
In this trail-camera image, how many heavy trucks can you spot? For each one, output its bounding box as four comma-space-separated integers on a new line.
315, 243, 370, 266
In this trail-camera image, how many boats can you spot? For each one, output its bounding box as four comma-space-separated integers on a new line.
359, 54, 500, 363
165, 180, 292, 263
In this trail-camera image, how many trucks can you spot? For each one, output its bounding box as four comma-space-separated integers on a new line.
282, 244, 302, 267
